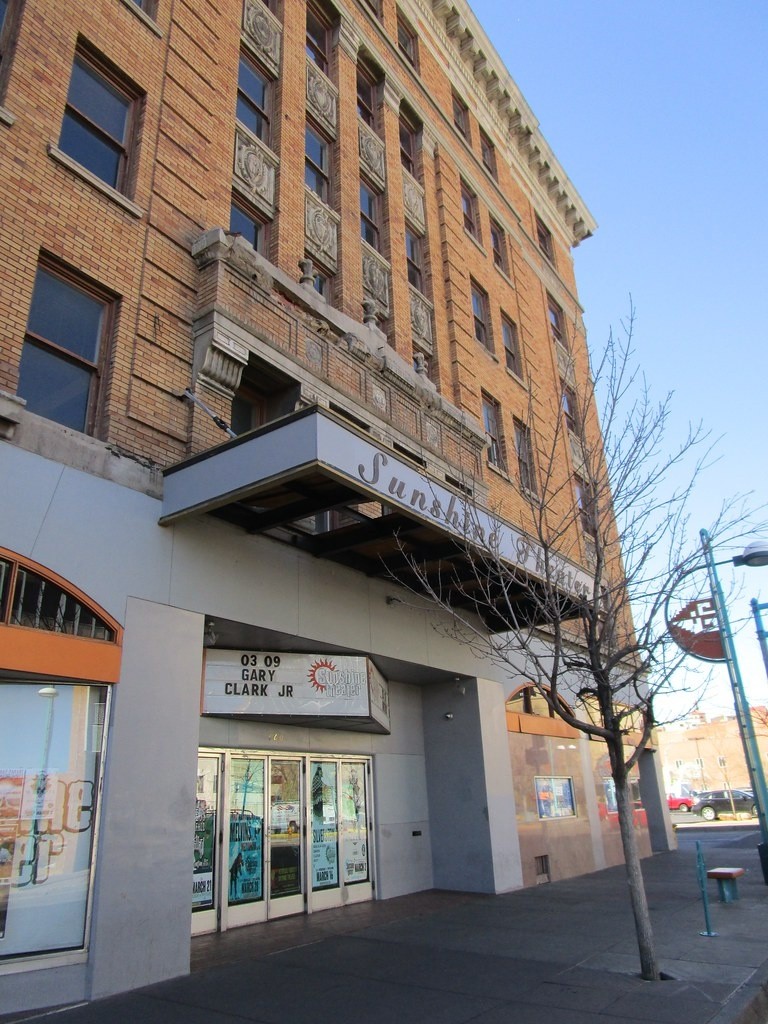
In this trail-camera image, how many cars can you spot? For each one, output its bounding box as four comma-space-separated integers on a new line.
692, 789, 758, 821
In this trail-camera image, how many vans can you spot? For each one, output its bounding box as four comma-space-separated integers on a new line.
261, 800, 336, 834
665, 783, 694, 812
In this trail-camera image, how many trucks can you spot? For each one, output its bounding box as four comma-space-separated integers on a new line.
196, 808, 263, 871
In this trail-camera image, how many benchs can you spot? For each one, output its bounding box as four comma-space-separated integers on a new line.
707, 867, 746, 903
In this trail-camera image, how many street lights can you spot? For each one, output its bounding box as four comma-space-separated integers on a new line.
16, 684, 59, 886
699, 529, 768, 880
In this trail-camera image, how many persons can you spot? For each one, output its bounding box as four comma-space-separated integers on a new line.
230, 853, 244, 900
